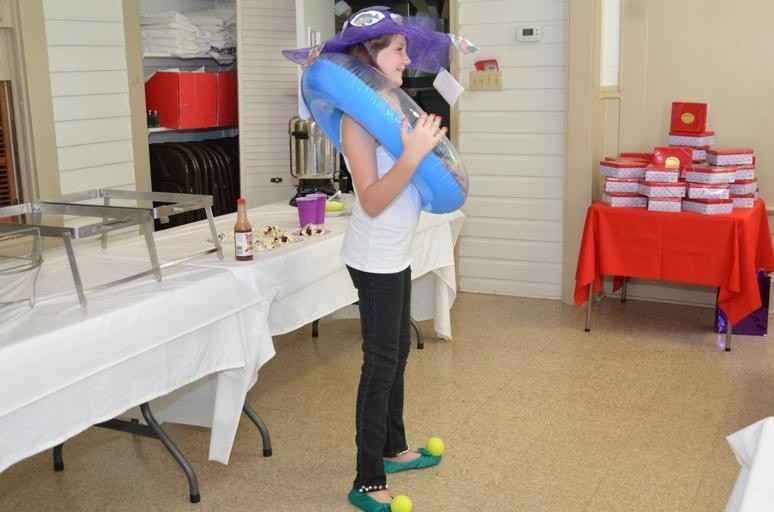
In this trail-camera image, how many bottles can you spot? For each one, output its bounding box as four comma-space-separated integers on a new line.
235, 198, 256, 262
150, 110, 161, 127
147, 108, 153, 128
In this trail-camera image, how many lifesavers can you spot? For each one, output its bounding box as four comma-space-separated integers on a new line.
300, 54, 469, 214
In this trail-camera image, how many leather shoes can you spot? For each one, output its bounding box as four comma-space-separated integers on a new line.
346, 482, 413, 512
382, 437, 448, 475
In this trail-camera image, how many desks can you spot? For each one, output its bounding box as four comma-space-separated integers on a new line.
574, 200, 774, 351
724, 416, 774, 511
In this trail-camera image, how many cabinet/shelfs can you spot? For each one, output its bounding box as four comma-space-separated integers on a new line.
334, 0, 459, 192
122, 0, 241, 235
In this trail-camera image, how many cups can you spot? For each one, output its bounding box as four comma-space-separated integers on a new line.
304, 192, 327, 231
295, 196, 317, 236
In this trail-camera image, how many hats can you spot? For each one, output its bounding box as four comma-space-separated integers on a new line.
280, 4, 457, 77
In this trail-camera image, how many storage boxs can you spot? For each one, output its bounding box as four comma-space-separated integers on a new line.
145, 70, 239, 131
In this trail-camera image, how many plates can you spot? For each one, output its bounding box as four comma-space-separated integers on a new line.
324, 211, 345, 219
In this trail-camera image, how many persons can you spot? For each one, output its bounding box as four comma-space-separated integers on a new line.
330, 3, 447, 512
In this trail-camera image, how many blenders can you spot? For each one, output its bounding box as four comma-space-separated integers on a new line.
284, 113, 350, 208
